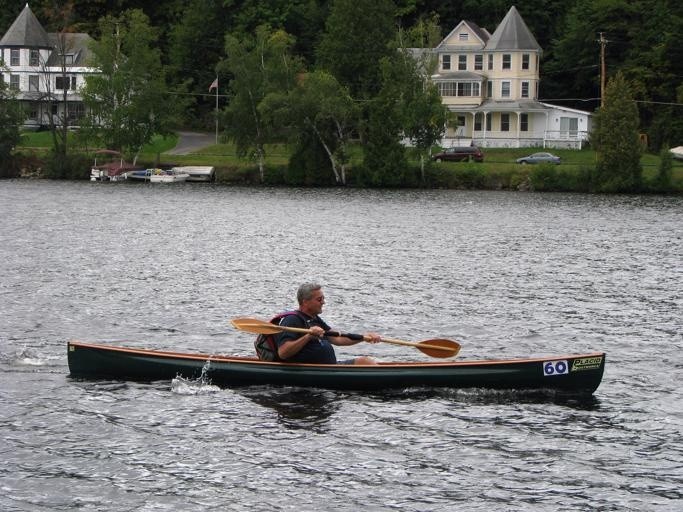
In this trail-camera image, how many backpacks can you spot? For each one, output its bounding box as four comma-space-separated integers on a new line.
252, 311, 310, 361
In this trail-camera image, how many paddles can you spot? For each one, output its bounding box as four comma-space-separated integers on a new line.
230, 318, 461, 358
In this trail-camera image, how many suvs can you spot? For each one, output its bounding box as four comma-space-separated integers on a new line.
430, 146, 484, 166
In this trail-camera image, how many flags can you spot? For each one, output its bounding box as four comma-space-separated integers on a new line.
208, 75, 218, 94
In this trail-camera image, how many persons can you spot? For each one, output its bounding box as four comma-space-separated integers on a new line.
272, 285, 381, 365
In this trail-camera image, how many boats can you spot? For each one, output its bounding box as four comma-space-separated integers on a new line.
64, 340, 610, 396
87, 147, 217, 189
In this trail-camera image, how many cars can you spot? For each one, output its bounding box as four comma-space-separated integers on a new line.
516, 152, 562, 168
667, 146, 683, 161
